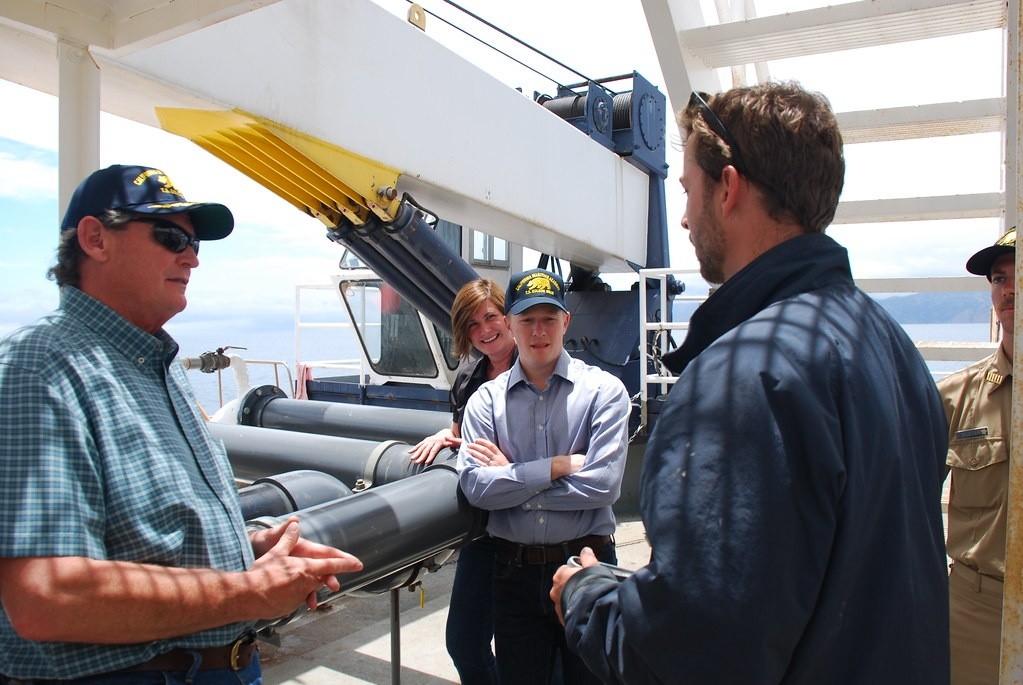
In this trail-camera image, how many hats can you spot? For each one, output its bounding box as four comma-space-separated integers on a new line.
966, 227, 1015, 276
61, 166, 234, 240
505, 268, 567, 315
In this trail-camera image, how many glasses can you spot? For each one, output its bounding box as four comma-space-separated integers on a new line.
683, 91, 746, 178
128, 217, 199, 253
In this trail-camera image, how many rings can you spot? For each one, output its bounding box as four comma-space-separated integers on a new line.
485, 458, 490, 464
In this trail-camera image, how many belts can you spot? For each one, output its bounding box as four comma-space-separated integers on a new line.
125, 634, 255, 670
497, 536, 610, 565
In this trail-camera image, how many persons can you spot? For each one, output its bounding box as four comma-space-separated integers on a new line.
0, 162, 364, 684
934, 225, 1016, 685
409, 278, 520, 684
457, 268, 633, 683
549, 82, 949, 683
